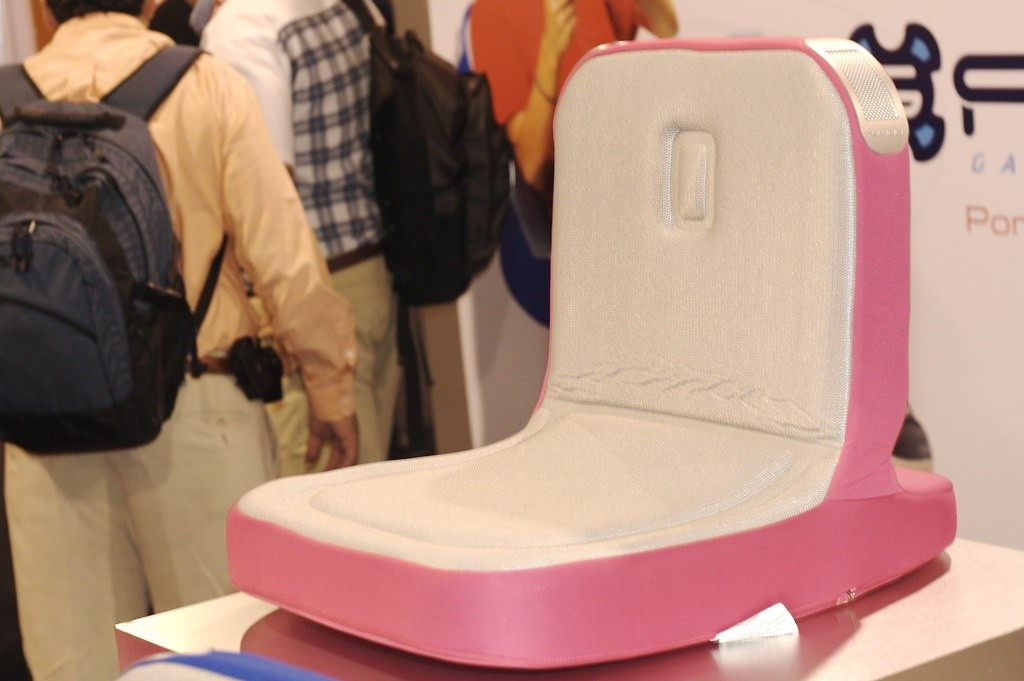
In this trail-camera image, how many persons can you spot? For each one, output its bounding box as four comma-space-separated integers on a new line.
190, 0, 400, 475
0, 0, 361, 681
471, 0, 678, 330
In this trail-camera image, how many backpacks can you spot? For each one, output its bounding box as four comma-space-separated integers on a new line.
0, 44, 231, 455
344, 0, 512, 306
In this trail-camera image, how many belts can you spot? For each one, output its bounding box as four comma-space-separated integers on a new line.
184, 355, 231, 376
244, 241, 390, 297
